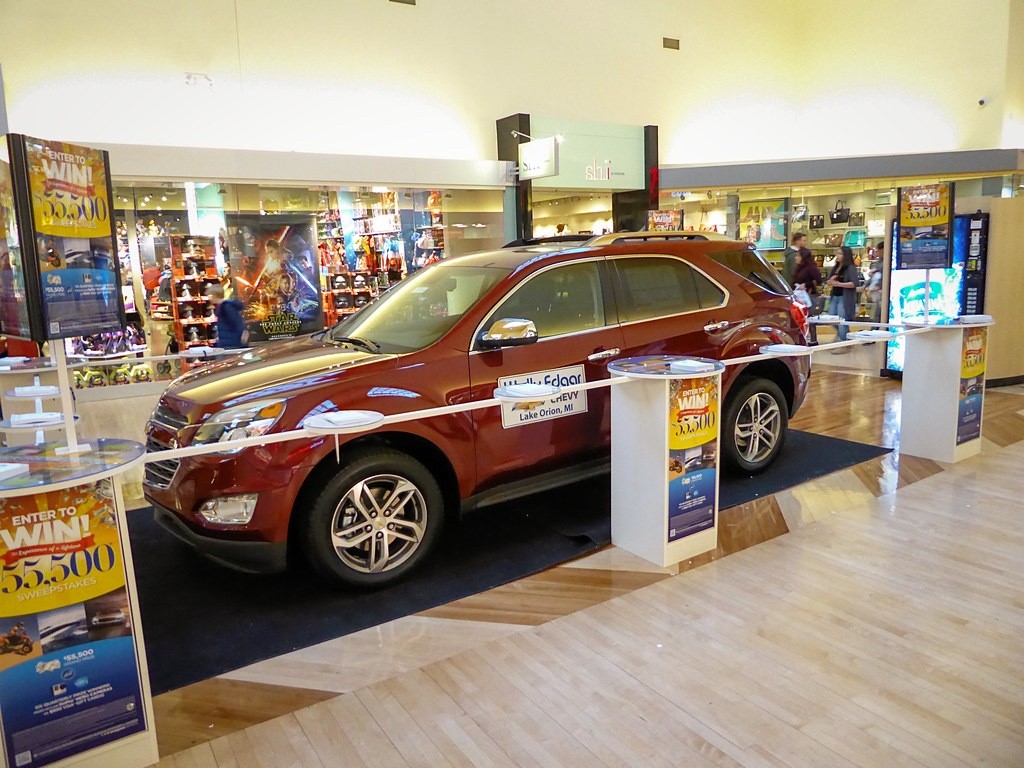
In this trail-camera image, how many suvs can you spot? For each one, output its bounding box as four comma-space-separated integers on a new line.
140, 227, 814, 592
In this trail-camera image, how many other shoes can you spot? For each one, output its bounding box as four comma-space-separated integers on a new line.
832, 347, 847, 354
863, 341, 876, 346
808, 341, 818, 347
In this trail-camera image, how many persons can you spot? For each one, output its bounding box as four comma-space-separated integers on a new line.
780, 232, 807, 285
207, 284, 250, 360
862, 242, 883, 331
791, 247, 822, 346
826, 246, 859, 355
266, 240, 313, 306
9, 235, 56, 336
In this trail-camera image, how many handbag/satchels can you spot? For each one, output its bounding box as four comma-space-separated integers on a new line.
793, 283, 812, 307
809, 200, 878, 297
807, 294, 826, 316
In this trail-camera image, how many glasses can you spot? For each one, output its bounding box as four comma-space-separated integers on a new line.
185, 244, 203, 249
